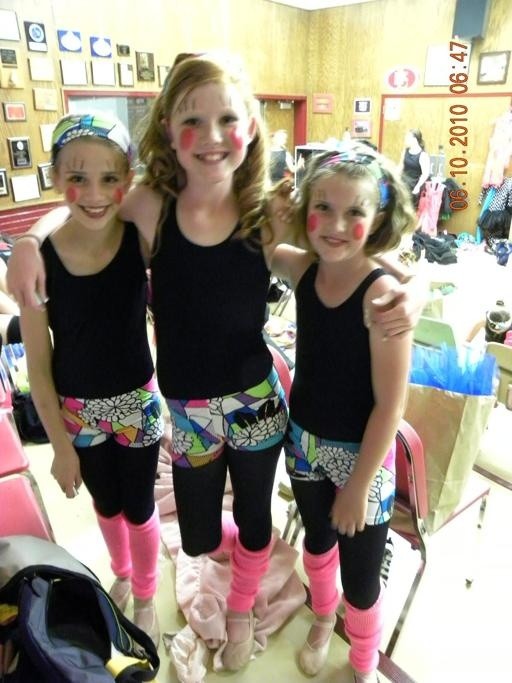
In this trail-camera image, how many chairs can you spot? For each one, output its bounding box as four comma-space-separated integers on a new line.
262, 341, 305, 540
0, 470, 58, 543
0, 410, 30, 477
0, 378, 15, 410
412, 315, 457, 354
482, 341, 512, 412
290, 418, 491, 660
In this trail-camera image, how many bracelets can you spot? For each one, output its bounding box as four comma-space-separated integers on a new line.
17, 233, 42, 248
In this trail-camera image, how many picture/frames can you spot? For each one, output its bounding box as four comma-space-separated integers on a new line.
0, 8, 171, 203
475, 50, 512, 84
350, 117, 374, 139
311, 92, 334, 114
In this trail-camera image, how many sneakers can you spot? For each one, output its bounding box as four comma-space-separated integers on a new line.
109, 574, 132, 614
222, 608, 257, 674
297, 609, 339, 677
132, 600, 159, 654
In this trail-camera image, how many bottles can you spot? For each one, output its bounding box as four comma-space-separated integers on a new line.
484, 300, 512, 344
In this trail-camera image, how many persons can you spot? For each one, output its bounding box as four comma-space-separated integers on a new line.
6, 53, 433, 673
18, 108, 169, 653
268, 145, 416, 683
398, 128, 431, 215
264, 129, 306, 186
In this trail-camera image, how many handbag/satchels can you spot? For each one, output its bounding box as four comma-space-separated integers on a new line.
11, 389, 51, 445
0, 533, 162, 682
